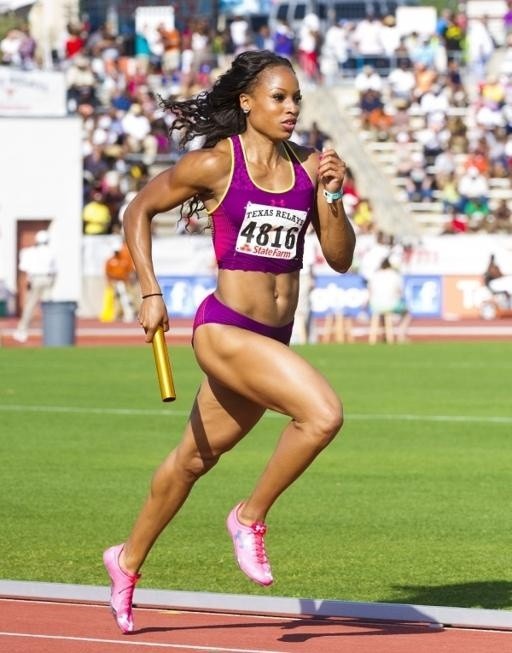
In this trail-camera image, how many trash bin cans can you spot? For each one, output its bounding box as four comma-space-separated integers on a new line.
42, 302, 77, 347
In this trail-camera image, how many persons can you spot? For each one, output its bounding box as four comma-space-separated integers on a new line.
7, 230, 63, 344
107, 250, 138, 321
485, 255, 511, 299
0, 26, 23, 69
62, 0, 512, 237
18, 27, 38, 70
297, 232, 410, 341
101, 48, 356, 635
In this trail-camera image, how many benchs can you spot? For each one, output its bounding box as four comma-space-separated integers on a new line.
335, 84, 512, 235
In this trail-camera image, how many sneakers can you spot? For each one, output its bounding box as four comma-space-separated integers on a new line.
103, 543, 142, 634
226, 501, 273, 587
